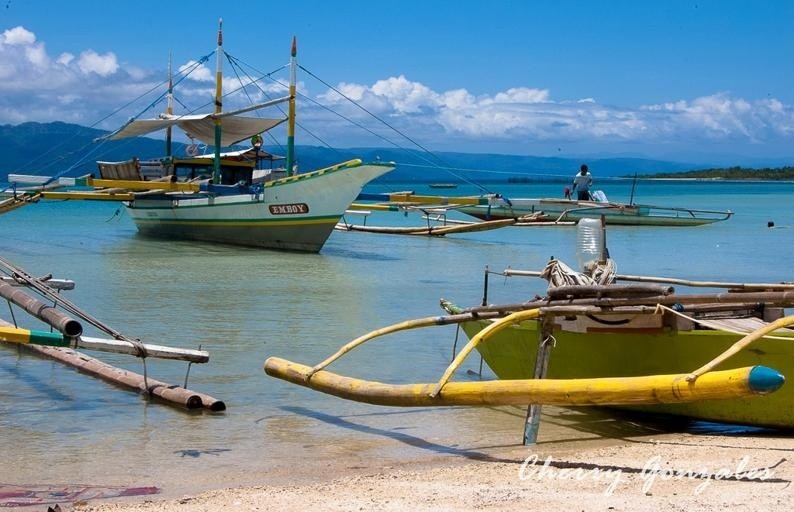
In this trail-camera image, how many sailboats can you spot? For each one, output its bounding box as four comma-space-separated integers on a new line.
102, 16, 396, 254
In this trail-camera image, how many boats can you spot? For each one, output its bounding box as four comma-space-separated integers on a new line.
439, 296, 793, 429
429, 183, 458, 190
446, 199, 733, 227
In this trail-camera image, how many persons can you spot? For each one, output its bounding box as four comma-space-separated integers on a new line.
570, 164, 593, 201
666, 287, 675, 295
670, 304, 684, 312
